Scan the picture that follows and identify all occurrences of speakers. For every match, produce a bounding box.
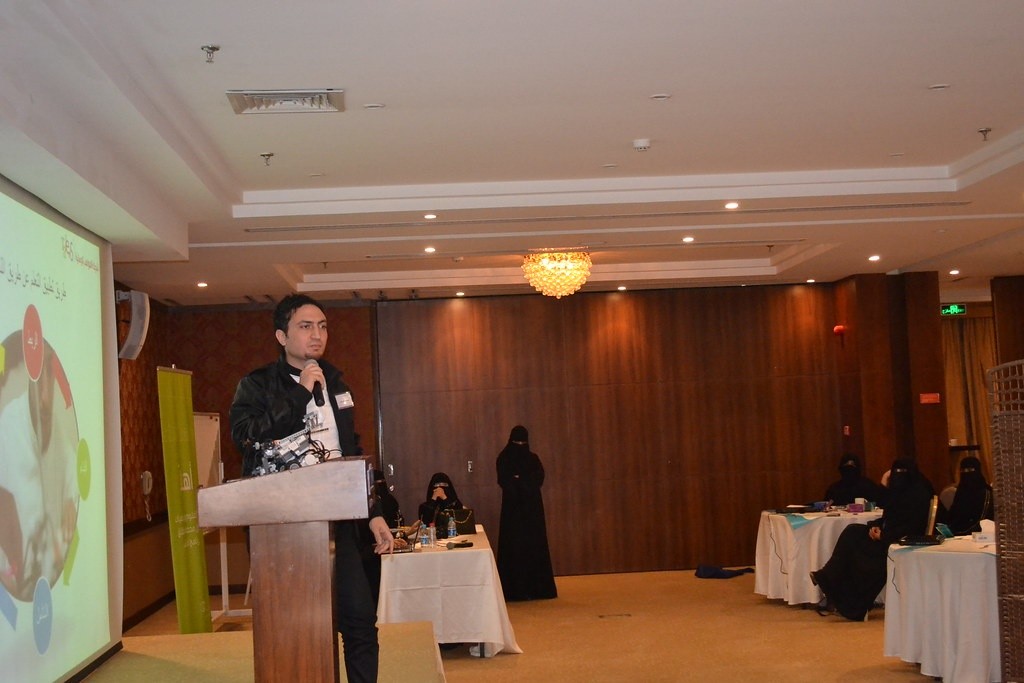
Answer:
[118,290,150,359]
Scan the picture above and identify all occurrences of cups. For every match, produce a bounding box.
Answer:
[951,439,957,446]
[854,498,864,504]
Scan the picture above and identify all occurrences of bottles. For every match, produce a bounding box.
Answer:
[428,523,436,548]
[448,517,456,538]
[420,525,429,551]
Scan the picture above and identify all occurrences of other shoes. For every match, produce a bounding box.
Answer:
[811,570,820,587]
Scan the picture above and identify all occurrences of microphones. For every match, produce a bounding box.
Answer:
[304,358,326,406]
[446,541,474,550]
[415,514,424,547]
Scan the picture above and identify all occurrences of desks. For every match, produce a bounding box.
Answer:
[375,524,523,657]
[883,532,1002,683]
[755,504,885,606]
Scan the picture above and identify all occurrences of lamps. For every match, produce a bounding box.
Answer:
[521,247,593,300]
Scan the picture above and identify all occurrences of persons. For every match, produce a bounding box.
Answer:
[496,426,558,601]
[945,456,995,537]
[828,455,886,505]
[418,473,473,651]
[229,293,396,683]
[370,469,403,529]
[810,459,937,620]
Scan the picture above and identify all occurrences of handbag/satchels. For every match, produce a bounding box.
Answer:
[443,508,477,537]
